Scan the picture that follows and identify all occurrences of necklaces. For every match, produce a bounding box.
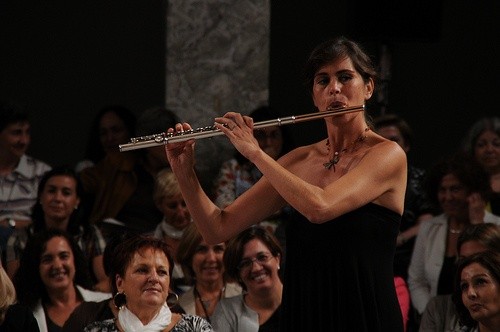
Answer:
[447,227,466,234]
[196,288,222,325]
[324,127,369,168]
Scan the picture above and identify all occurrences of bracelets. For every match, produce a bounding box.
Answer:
[398,233,406,246]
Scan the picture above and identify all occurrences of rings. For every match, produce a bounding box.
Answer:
[231,125,237,131]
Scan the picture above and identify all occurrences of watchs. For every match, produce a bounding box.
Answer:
[5,218,17,231]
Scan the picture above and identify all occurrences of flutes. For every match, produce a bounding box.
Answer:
[118,104,366,153]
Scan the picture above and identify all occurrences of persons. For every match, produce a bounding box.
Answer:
[165,38,408,332]
[0,107,500,332]
